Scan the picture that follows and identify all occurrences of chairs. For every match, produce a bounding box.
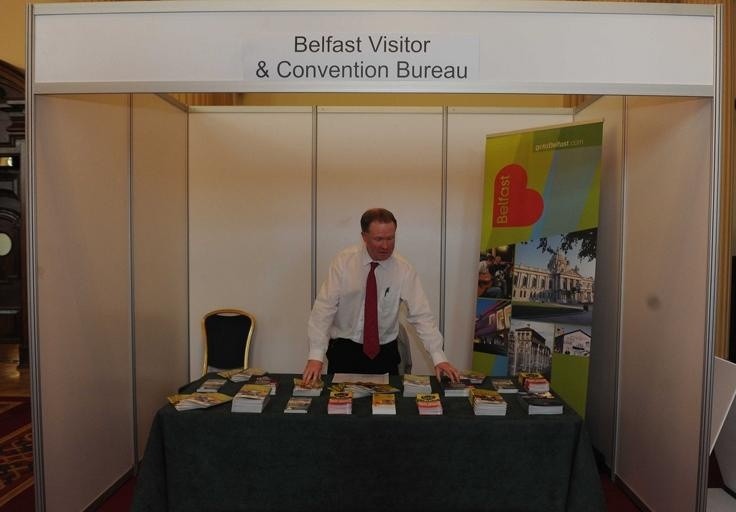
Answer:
[176,308,258,395]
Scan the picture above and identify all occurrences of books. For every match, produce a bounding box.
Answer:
[165,367,565,415]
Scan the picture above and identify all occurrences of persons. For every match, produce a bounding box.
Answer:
[301,209,461,388]
[479,254,512,299]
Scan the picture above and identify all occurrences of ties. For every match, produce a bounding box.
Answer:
[363,262,381,360]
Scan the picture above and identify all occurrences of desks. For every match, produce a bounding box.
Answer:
[155,376,583,512]
